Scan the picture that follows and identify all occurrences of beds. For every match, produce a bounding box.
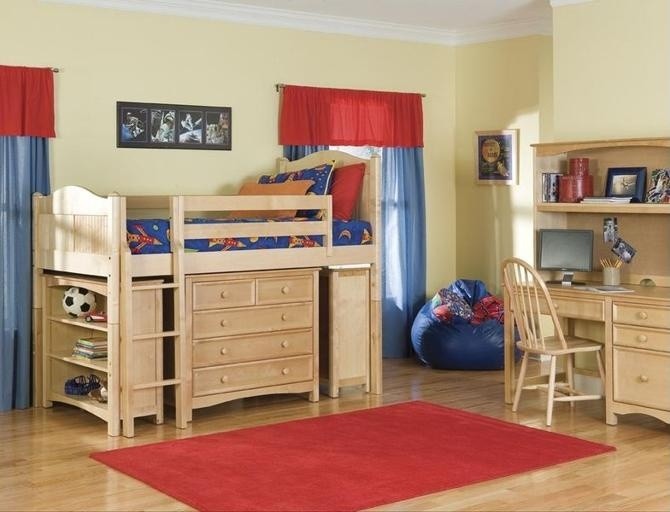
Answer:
[29,150,382,438]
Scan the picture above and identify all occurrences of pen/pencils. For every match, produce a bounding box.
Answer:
[599,256,624,269]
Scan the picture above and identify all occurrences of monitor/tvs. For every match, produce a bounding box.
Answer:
[538,228,594,287]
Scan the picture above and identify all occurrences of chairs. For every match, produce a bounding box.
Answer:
[499,256,606,425]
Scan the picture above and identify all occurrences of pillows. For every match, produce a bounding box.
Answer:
[225,159,367,222]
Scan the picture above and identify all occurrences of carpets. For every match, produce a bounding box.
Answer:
[86,400,617,512]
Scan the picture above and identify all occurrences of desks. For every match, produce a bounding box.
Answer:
[500,281,669,431]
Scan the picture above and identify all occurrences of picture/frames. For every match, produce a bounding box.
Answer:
[474,129,520,186]
[605,166,647,205]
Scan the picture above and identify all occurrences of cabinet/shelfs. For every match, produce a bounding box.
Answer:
[529,136,670,215]
[34,271,162,438]
[164,265,323,415]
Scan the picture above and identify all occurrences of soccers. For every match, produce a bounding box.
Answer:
[62,286,99,320]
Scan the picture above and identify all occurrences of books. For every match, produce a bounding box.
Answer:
[580,195,632,204]
[574,282,634,298]
[542,172,564,202]
[70,337,108,364]
